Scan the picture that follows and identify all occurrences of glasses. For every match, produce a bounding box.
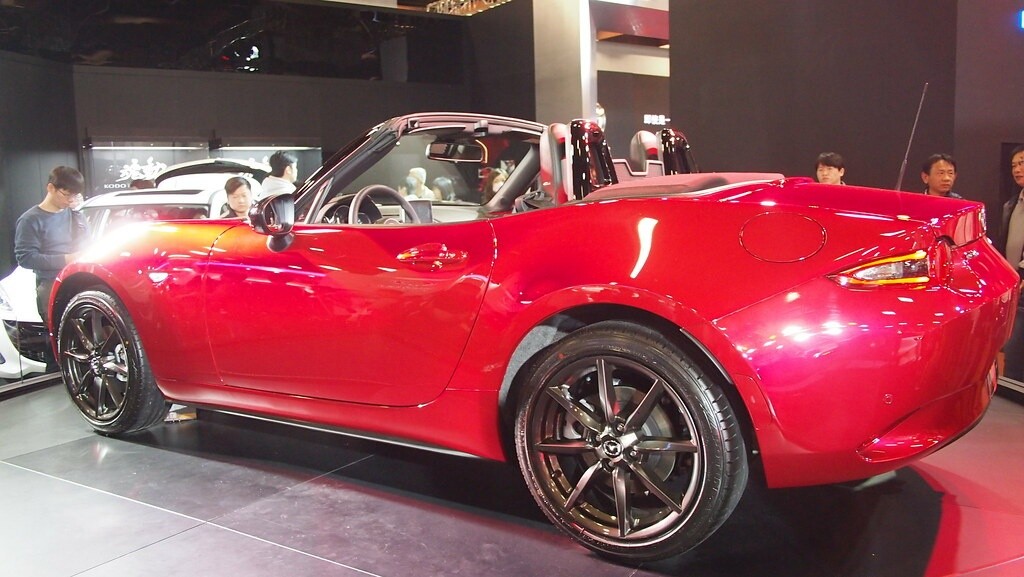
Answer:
[57,186,79,198]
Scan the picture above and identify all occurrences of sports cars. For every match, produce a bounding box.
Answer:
[44,109,1022,567]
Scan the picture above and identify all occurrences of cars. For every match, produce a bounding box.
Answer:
[0,158,272,382]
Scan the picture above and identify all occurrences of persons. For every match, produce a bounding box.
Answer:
[219,177,254,218]
[999,145,1024,298]
[257,150,298,200]
[921,153,963,199]
[129,179,153,191]
[397,167,462,201]
[815,152,846,185]
[14,165,88,372]
[485,167,510,202]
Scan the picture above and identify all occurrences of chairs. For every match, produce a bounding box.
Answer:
[514,118,619,211]
[630,128,699,176]
